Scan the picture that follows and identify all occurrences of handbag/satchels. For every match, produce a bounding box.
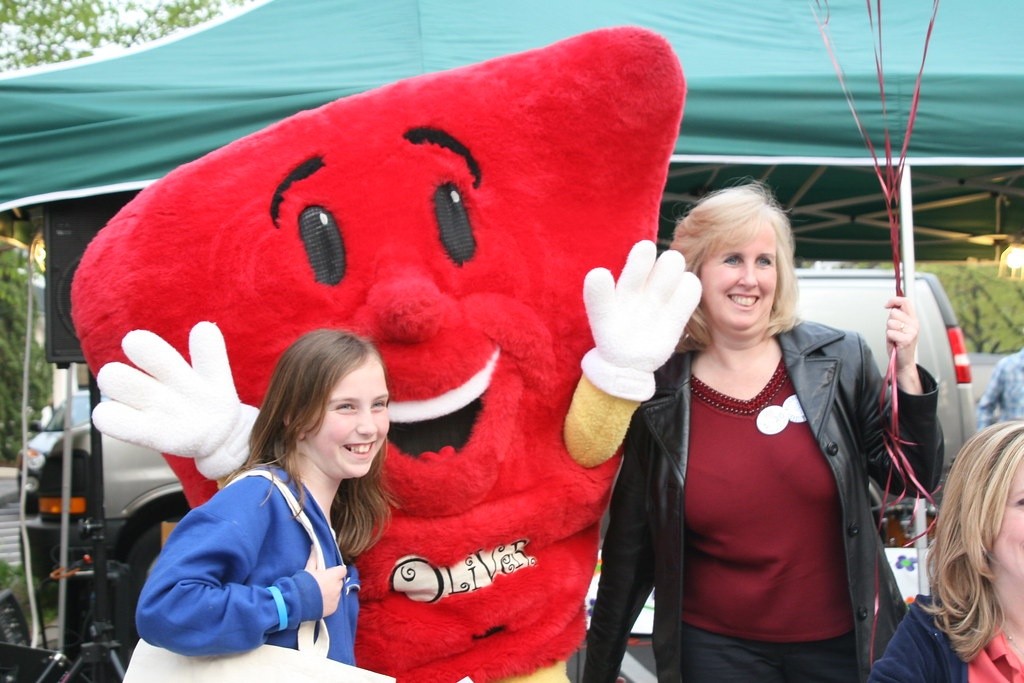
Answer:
[120,469,396,683]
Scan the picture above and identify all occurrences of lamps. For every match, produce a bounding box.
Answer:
[996,241,1024,282]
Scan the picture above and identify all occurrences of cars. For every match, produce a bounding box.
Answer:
[16,386,189,632]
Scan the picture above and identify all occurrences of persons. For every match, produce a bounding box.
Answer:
[579,183,945,683]
[976,346,1024,432]
[135,328,401,667]
[868,419,1024,683]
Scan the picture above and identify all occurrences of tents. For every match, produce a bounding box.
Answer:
[0,0,1024,652]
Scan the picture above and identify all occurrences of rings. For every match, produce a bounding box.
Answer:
[899,323,904,332]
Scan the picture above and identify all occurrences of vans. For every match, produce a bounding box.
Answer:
[789,268,982,515]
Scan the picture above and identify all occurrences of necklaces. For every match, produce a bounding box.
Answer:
[1002,628,1024,657]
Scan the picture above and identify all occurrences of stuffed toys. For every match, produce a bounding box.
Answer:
[71,25,702,683]
